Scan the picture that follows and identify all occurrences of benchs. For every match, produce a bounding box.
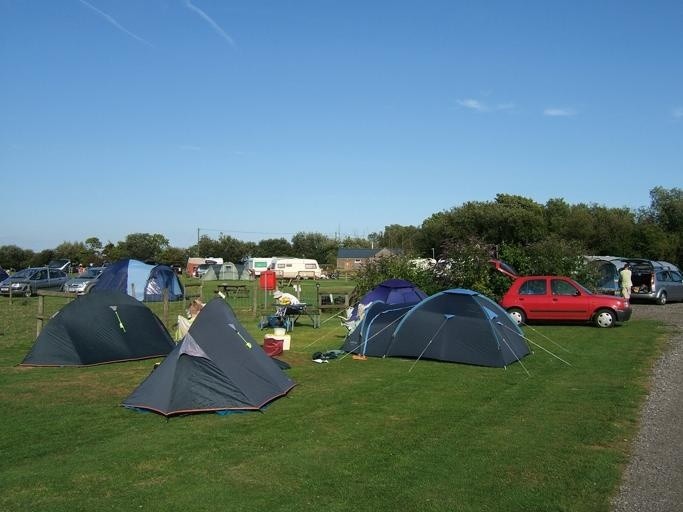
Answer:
[214,290,250,296]
[271,290,354,329]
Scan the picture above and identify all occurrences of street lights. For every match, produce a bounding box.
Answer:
[431,247,434,259]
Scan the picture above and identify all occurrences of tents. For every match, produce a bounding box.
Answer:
[120,293,298,416]
[92,257,184,303]
[340,286,531,369]
[336,275,429,334]
[12,288,177,368]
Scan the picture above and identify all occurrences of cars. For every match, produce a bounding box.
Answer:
[486,259,632,329]
[0,258,107,298]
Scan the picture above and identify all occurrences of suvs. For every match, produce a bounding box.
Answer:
[620,256,682,305]
[170,264,181,275]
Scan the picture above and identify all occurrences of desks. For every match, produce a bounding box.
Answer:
[217,284,248,298]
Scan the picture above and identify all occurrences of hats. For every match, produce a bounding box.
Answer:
[273,290,283,300]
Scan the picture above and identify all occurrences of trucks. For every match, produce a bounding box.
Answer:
[194,255,322,281]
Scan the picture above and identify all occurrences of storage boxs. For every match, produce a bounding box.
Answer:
[263,333,291,351]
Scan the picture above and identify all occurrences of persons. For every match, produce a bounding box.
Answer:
[270,288,301,313]
[619,263,634,304]
